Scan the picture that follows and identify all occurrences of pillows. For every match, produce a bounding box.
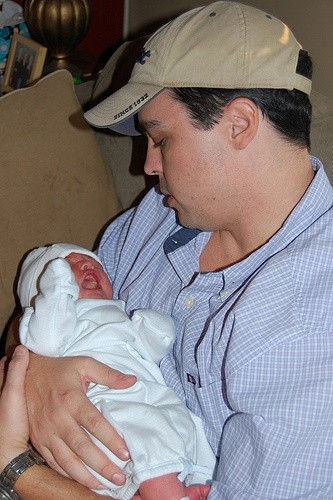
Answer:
[0,69,124,359]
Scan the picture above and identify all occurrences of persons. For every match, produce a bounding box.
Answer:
[17,242,215,500]
[0,0,333,500]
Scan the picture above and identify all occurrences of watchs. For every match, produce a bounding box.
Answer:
[0,448,46,500]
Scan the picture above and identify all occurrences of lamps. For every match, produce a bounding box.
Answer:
[24,0,99,72]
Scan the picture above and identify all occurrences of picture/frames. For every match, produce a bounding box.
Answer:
[0,33,47,94]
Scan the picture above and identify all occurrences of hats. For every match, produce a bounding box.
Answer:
[17,242,103,307]
[83,1,312,136]
